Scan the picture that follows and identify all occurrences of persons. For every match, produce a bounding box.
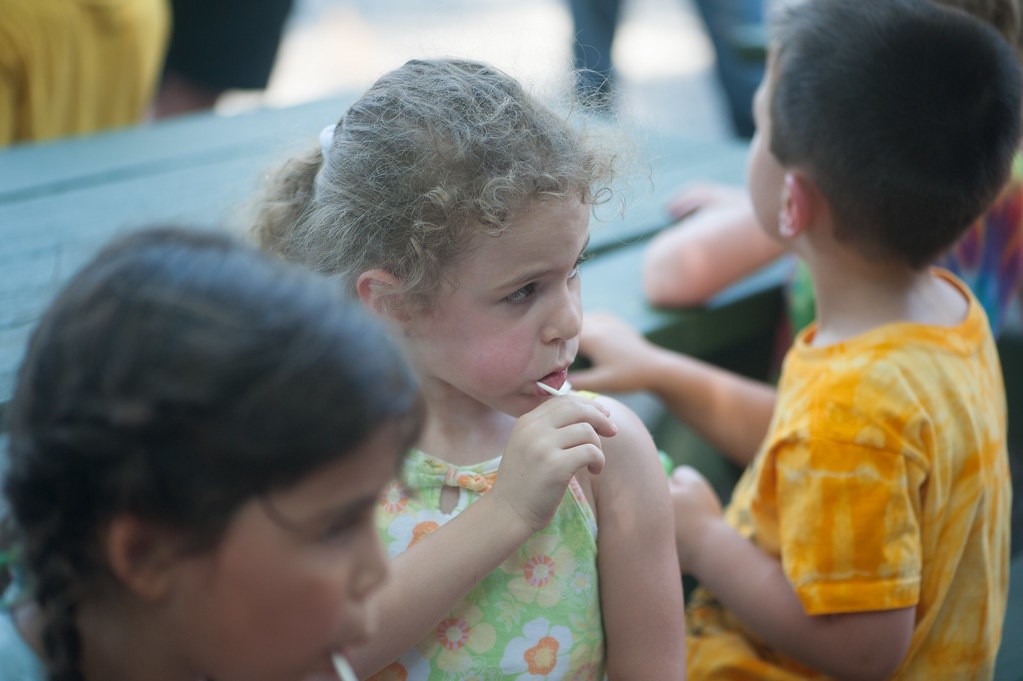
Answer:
[572,0,1023,681]
[573,0,769,139]
[1,228,431,680]
[640,0,1023,350]
[254,54,686,681]
